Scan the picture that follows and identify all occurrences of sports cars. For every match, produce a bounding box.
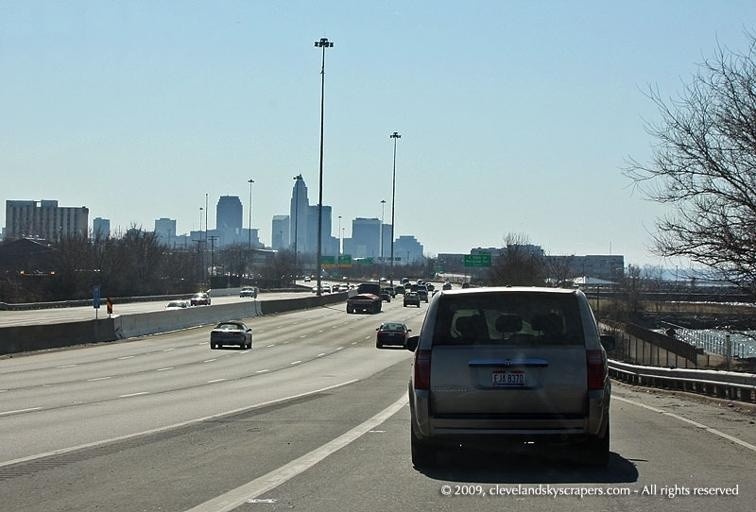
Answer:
[210,320,254,351]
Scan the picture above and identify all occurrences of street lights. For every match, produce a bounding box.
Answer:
[337,215,341,255]
[388,132,403,272]
[200,207,203,241]
[380,200,386,258]
[312,38,335,282]
[199,191,209,249]
[341,227,345,254]
[293,175,303,286]
[247,179,255,249]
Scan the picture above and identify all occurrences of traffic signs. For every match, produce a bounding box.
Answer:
[463,254,492,268]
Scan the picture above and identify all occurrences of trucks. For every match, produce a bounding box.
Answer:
[346,283,382,314]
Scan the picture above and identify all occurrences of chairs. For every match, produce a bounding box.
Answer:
[451,311,581,344]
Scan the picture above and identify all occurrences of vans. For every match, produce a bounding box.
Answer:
[400,282,611,472]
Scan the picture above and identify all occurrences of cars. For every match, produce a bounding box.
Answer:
[190,293,212,306]
[379,277,478,308]
[304,276,311,282]
[165,300,190,310]
[240,286,258,298]
[375,321,412,348]
[312,274,352,293]
